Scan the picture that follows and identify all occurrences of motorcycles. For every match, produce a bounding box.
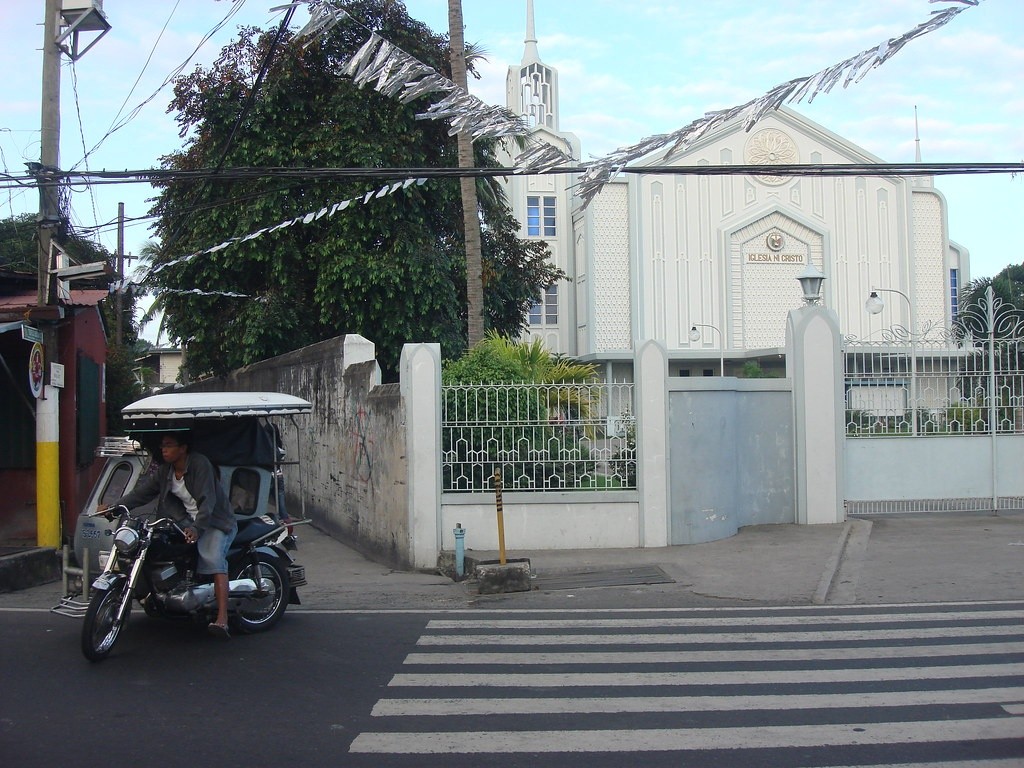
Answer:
[51,389,312,664]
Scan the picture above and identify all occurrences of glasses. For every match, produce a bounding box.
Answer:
[159,443,180,450]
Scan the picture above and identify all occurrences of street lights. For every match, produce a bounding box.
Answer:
[688,322,725,377]
[865,286,913,339]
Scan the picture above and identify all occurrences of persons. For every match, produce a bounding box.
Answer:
[265,423,289,521]
[98,429,238,639]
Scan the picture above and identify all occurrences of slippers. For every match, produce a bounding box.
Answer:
[208,623,232,639]
